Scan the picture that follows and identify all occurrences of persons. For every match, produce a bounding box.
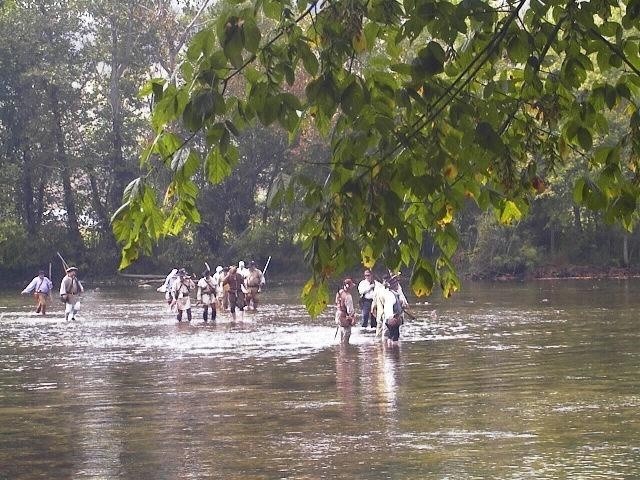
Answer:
[59,267,83,321]
[162,258,267,322]
[335,279,355,346]
[358,268,409,346]
[20,269,53,315]
